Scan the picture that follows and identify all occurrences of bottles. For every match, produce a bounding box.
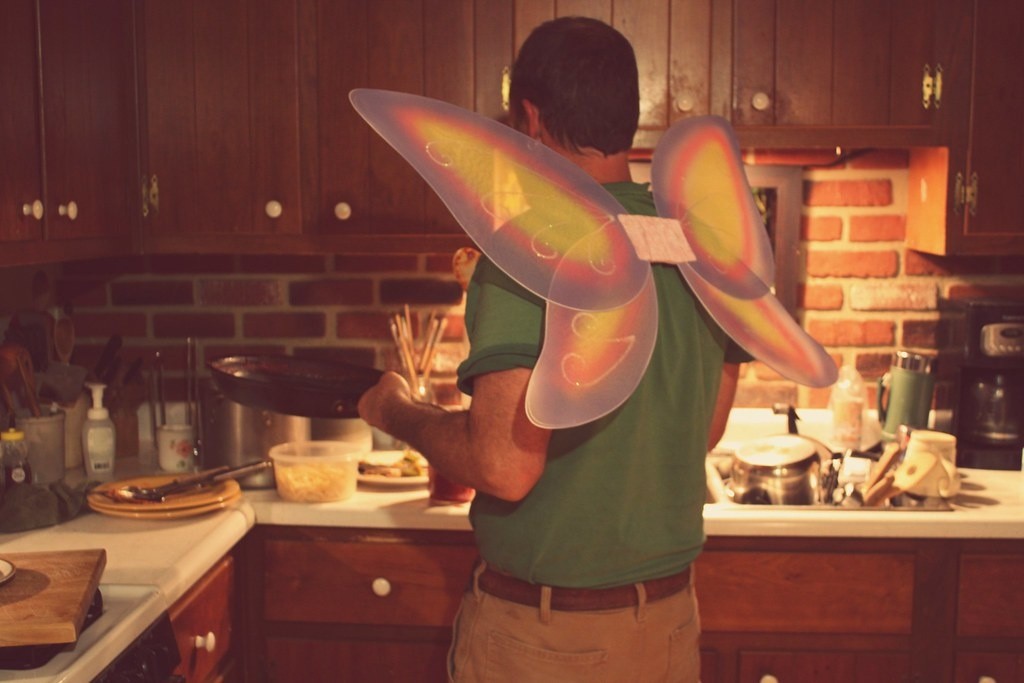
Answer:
[831,352,864,451]
[0,427,32,486]
[427,384,473,506]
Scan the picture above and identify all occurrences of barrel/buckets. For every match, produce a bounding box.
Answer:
[198,378,373,490]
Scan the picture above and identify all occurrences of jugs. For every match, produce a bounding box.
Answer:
[962,371,1024,447]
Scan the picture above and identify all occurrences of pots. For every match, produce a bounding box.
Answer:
[205,353,450,419]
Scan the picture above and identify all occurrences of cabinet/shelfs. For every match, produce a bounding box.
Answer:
[89,523,1024,683]
[0,0,1024,266]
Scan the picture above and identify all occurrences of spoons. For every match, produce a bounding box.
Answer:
[114,459,271,505]
[54,314,76,368]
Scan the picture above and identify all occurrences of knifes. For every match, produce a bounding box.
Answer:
[92,334,144,384]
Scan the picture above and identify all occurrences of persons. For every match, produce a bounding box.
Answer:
[357,16,755,683]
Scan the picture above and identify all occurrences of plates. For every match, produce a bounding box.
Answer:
[358,449,430,484]
[0,557,16,585]
[86,474,241,519]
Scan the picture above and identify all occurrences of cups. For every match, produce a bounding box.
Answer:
[892,424,961,499]
[725,435,822,505]
[15,411,66,483]
[158,423,196,472]
[49,400,87,467]
[875,350,937,436]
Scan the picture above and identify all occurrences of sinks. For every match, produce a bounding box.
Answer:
[702,459,729,503]
[704,444,956,516]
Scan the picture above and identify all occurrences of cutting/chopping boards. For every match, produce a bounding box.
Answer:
[0,548,107,647]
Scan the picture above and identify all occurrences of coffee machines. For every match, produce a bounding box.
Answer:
[929,296,1024,471]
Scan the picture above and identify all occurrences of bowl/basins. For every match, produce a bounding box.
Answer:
[267,440,360,503]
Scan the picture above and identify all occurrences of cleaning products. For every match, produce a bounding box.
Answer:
[84,382,117,480]
